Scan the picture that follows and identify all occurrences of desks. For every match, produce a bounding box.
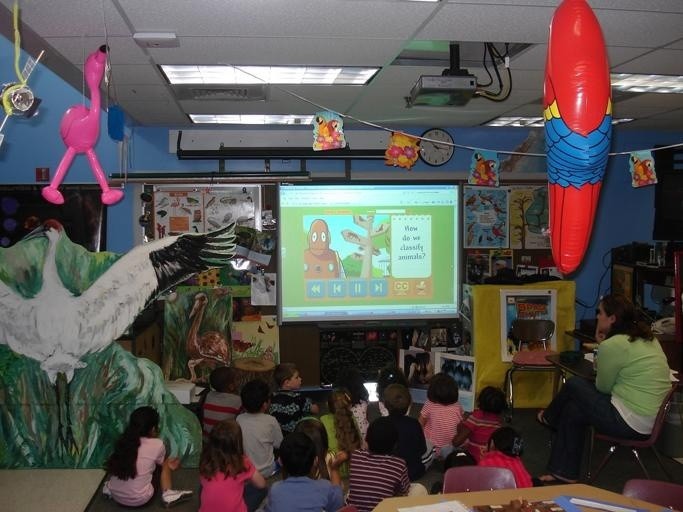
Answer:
[277,363,335,401]
[370,483,683,512]
[0,469,109,512]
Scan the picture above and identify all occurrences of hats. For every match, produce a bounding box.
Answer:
[494,427,522,456]
[442,447,474,468]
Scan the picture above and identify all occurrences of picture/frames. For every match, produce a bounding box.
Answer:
[509,186,552,250]
[462,183,510,249]
[500,288,557,364]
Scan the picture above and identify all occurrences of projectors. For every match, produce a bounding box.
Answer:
[409,68,478,108]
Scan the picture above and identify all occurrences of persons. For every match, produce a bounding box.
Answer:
[362,384,426,484]
[201,367,246,441]
[102,406,193,509]
[319,386,366,484]
[343,416,411,512]
[266,432,348,512]
[478,427,534,489]
[265,362,319,438]
[198,419,266,512]
[416,373,464,458]
[452,386,509,465]
[294,419,340,485]
[338,373,371,451]
[235,378,287,481]
[366,365,420,421]
[532,293,669,484]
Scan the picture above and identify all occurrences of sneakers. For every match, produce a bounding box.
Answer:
[101,482,111,498]
[163,490,194,506]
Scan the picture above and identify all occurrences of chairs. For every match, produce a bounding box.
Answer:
[504,319,566,424]
[587,383,678,482]
[622,478,683,512]
[443,466,517,493]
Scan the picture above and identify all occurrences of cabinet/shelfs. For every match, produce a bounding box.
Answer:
[318,319,463,388]
[634,260,675,319]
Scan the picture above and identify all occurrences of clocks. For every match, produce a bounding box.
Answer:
[417,127,455,167]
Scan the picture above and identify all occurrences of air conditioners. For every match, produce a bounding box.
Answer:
[170,83,270,102]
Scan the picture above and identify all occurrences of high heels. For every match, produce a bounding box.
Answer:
[536,408,557,433]
